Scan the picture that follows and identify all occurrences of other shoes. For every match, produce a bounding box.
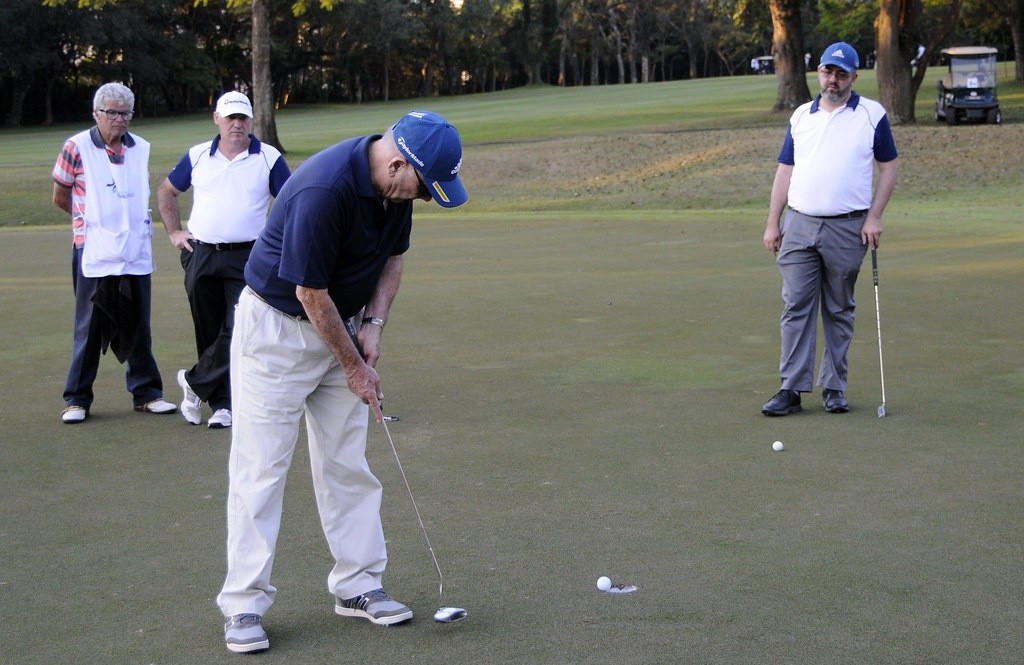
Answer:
[177,369,204,425]
[207,408,232,428]
[134,398,177,414]
[60,405,90,424]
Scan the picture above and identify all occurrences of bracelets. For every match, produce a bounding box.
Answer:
[360,316,385,329]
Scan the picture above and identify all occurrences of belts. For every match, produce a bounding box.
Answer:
[817,209,870,218]
[192,240,256,251]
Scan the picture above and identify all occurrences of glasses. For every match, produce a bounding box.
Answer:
[99,109,134,121]
[412,168,432,202]
[821,68,850,80]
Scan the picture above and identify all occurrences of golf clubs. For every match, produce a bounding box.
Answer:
[871,242,886,419]
[343,312,470,624]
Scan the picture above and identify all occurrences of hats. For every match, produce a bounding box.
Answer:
[392,109,470,208]
[216,91,254,119]
[818,42,860,73]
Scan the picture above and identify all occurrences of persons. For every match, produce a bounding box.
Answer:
[761,42,899,415]
[217,109,468,652]
[50,83,176,423]
[158,91,290,427]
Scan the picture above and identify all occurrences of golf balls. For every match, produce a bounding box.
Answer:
[596,575,611,592]
[773,440,784,451]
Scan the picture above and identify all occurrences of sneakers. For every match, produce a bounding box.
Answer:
[822,388,850,413]
[335,588,414,625]
[224,613,269,652]
[761,389,802,415]
